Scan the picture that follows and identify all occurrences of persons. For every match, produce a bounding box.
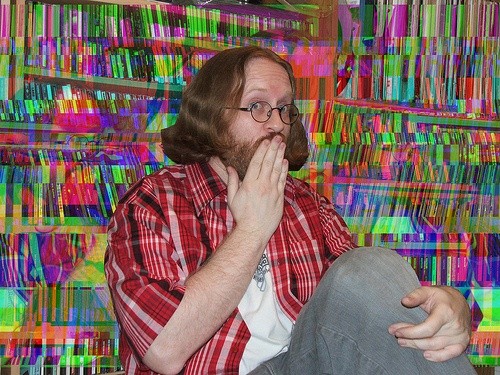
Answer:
[104,46,483,375]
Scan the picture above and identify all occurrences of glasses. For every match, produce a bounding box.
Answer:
[224,101,299,125]
